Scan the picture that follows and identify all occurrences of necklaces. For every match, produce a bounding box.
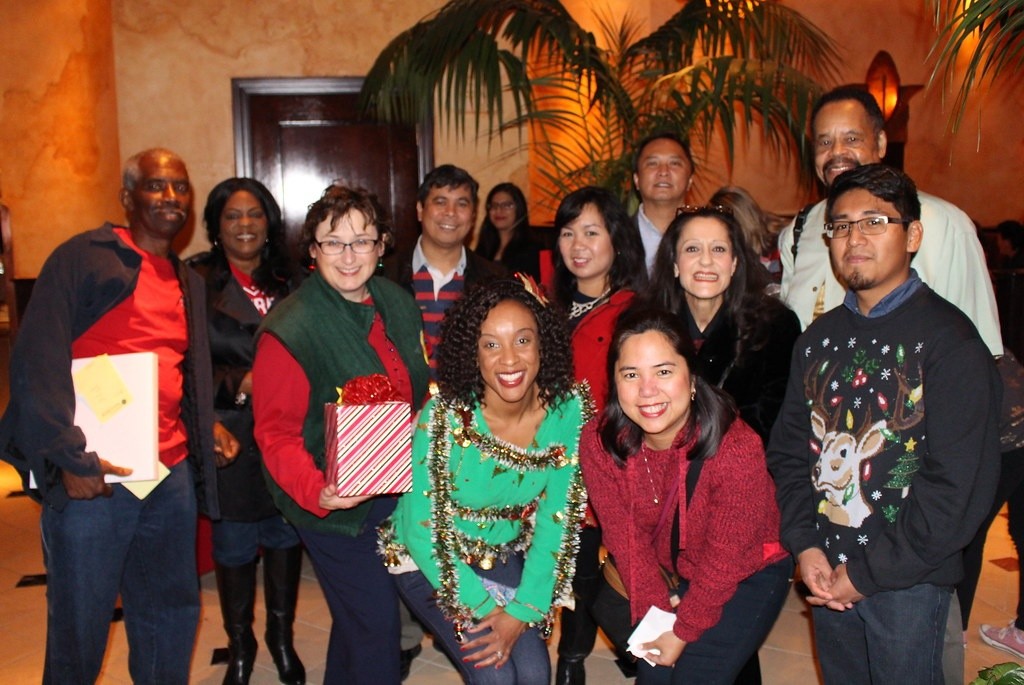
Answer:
[641,436,659,504]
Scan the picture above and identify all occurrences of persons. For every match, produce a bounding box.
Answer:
[0,83,1024,685]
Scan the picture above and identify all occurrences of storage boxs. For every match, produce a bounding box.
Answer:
[26,351,161,490]
[324,401,414,497]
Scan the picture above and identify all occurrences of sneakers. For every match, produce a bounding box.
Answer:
[978,617,1024,660]
[963,631,967,648]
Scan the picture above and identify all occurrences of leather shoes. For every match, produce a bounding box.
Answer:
[556,655,585,685]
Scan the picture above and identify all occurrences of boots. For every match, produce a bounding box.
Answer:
[213,555,260,685]
[261,541,306,685]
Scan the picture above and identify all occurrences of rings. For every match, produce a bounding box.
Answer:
[496,650,503,659]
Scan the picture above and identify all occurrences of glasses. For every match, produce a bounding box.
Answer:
[312,234,380,255]
[487,201,515,210]
[673,205,734,228]
[823,216,910,239]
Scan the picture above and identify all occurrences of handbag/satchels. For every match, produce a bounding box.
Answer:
[993,347,1024,453]
[591,550,681,663]
